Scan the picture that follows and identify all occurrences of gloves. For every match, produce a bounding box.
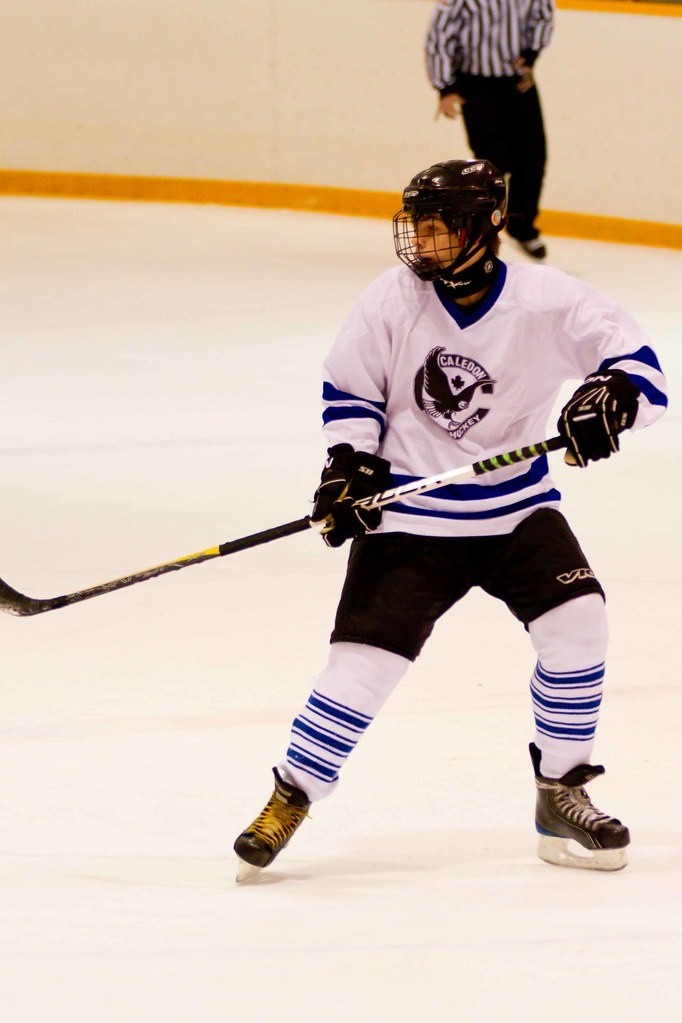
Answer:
[311,443,391,548]
[557,369,640,469]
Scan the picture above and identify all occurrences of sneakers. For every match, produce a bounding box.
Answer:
[529,742,630,870]
[234,766,312,883]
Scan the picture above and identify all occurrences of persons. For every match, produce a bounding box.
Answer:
[423,0,556,266]
[233,160,667,885]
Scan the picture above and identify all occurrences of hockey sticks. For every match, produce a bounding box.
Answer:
[0,435,566,617]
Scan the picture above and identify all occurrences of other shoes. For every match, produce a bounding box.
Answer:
[520,235,546,258]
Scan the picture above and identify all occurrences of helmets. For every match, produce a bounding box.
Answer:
[392,159,507,282]
